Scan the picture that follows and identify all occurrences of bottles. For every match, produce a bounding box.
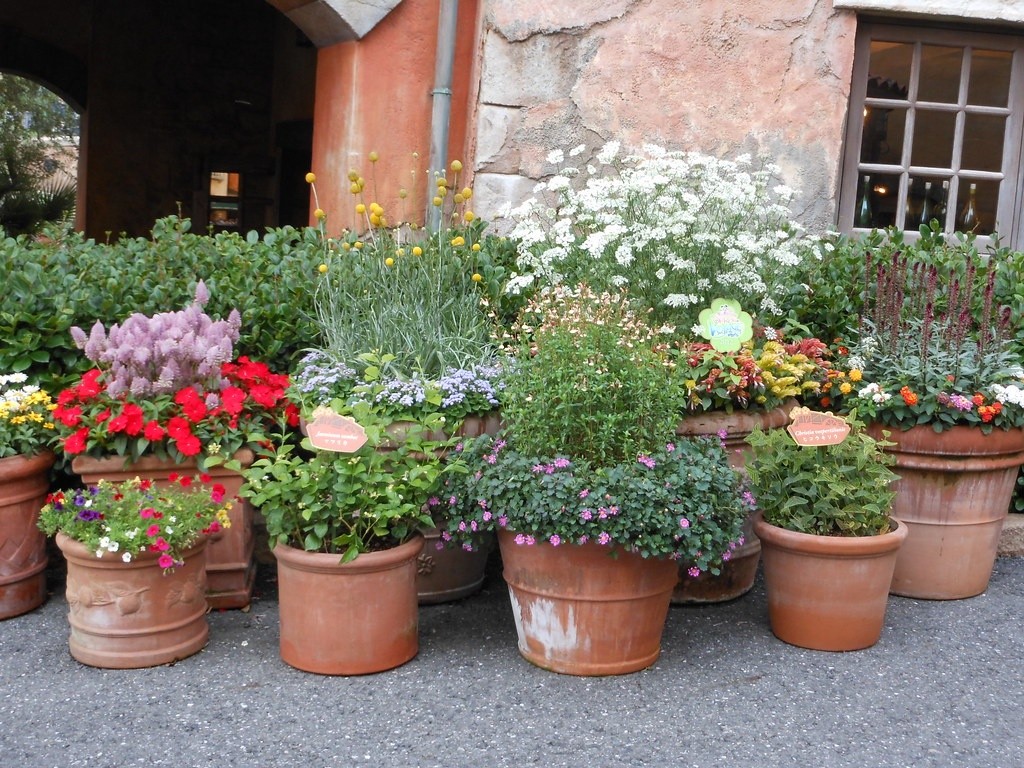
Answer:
[957,183,981,236]
[903,177,916,232]
[917,182,936,231]
[854,174,873,229]
[935,181,950,232]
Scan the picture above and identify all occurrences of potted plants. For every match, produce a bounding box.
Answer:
[744,407,908,654]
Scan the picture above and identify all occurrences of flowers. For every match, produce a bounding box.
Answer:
[0,372,58,460]
[47,278,299,472]
[653,322,859,409]
[36,468,243,576]
[826,249,1024,433]
[284,154,515,430]
[228,355,468,563]
[438,284,754,583]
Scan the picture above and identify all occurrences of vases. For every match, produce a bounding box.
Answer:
[859,422,1024,601]
[54,532,209,670]
[295,413,504,605]
[0,452,60,621]
[71,455,258,608]
[493,524,681,676]
[639,401,801,604]
[269,538,423,676]
